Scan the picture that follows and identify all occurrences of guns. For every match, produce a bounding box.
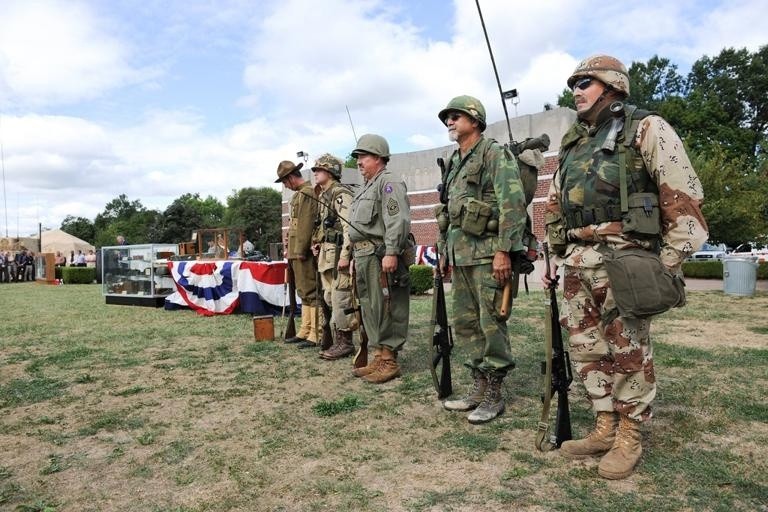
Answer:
[285,259,302,339]
[543,242,573,448]
[344,260,368,368]
[313,246,334,350]
[434,242,453,400]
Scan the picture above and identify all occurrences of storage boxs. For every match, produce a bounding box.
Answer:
[253,315,275,342]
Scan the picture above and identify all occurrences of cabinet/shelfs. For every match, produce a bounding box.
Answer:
[102,243,180,308]
[34,252,55,285]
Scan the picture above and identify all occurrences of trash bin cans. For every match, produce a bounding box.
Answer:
[720,255,760,296]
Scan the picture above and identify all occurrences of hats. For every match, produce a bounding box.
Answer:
[274,160,303,183]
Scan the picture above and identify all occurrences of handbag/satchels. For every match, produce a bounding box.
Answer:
[602,247,679,319]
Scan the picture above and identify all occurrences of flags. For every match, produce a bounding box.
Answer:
[412,245,453,273]
[537,240,545,257]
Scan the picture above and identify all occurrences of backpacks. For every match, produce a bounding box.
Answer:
[442,138,538,299]
[315,183,363,234]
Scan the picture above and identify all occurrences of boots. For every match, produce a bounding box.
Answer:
[322,327,355,360]
[350,348,381,377]
[361,348,401,383]
[444,378,488,411]
[598,414,642,479]
[318,328,342,358]
[468,380,504,423]
[559,411,619,459]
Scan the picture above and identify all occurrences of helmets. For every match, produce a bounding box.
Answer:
[311,155,342,183]
[351,134,391,161]
[438,96,487,132]
[567,55,630,97]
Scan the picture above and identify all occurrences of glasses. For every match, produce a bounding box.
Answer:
[444,114,460,124]
[573,76,591,90]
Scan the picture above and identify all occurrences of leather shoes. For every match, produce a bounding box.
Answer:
[296,339,316,348]
[284,337,302,343]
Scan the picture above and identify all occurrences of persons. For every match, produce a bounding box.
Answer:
[228,239,264,260]
[432,93,529,424]
[205,239,219,253]
[348,133,410,385]
[539,54,712,480]
[272,159,326,350]
[310,153,360,361]
[117,235,129,277]
[0,248,96,284]
